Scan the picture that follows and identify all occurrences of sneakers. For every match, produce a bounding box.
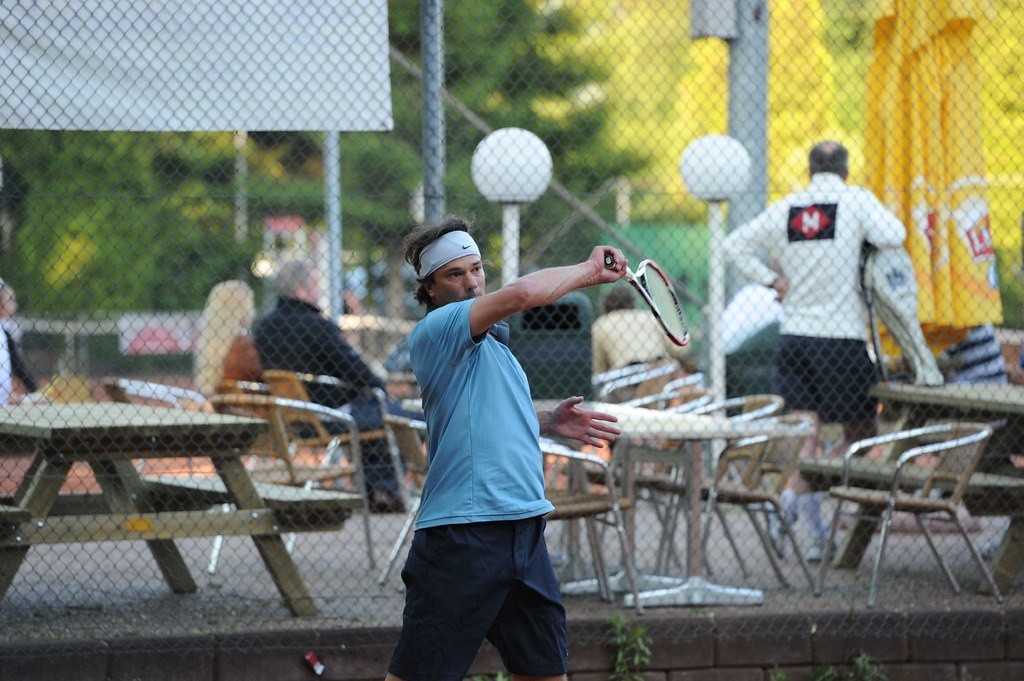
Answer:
[761,496,835,564]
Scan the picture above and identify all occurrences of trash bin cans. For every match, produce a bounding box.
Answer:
[502,290,593,401]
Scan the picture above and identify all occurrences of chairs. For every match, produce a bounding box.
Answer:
[0,356,1023,622]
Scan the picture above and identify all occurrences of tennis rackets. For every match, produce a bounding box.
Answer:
[604,254,691,345]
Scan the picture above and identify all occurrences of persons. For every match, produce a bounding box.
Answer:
[373,215,631,680]
[247,255,414,515]
[588,285,674,398]
[713,137,911,560]
[0,278,34,403]
